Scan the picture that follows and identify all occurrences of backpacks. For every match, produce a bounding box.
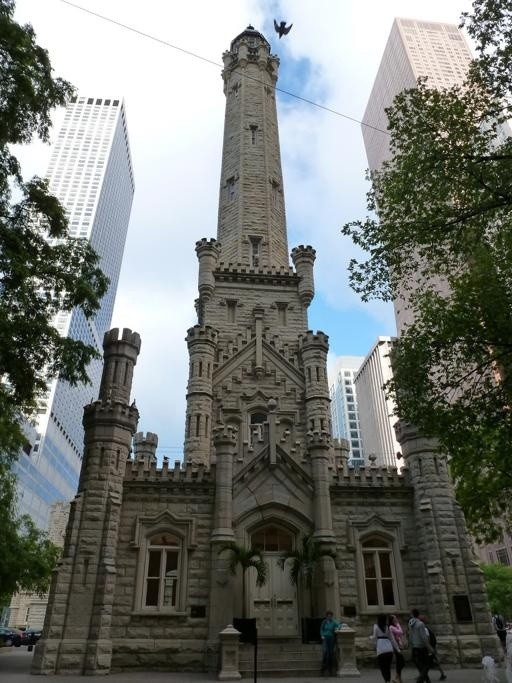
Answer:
[494,614,503,629]
[424,624,437,648]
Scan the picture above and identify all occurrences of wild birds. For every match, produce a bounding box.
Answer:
[274,19,293,39]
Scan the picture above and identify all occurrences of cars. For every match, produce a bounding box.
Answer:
[0,626,43,647]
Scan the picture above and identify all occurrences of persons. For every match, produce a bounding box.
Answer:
[407,607,436,682]
[368,612,401,683]
[416,614,447,680]
[388,614,406,682]
[319,609,342,676]
[492,609,507,655]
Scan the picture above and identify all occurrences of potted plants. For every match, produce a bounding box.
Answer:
[277,528,342,644]
[217,541,268,643]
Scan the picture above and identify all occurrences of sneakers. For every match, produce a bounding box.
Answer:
[383,675,447,683]
